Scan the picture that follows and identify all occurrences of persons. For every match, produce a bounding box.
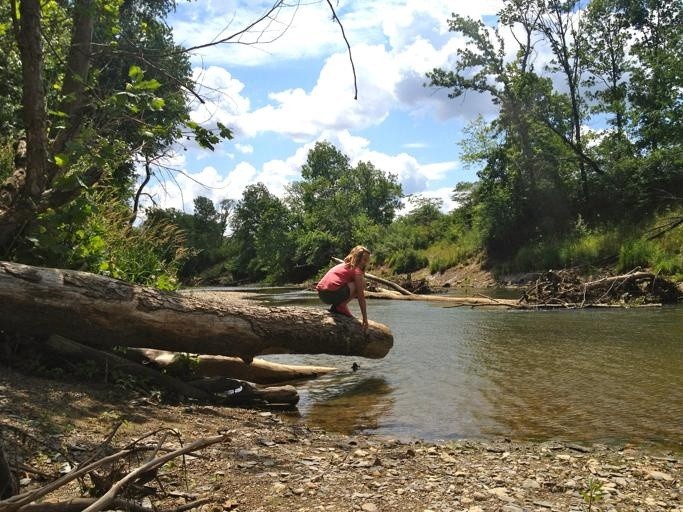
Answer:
[316,245,369,328]
